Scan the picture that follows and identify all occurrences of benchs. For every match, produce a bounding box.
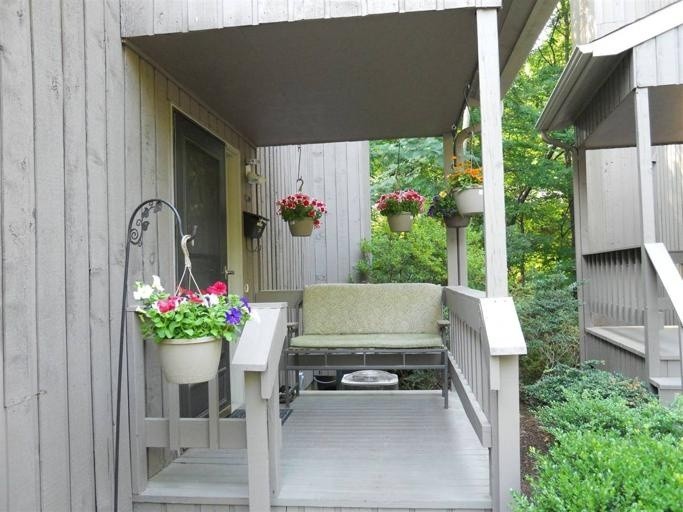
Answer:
[284,282,447,413]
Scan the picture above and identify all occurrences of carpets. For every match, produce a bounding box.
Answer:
[227,407,293,426]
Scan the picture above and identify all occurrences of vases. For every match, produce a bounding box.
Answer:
[450,183,485,215]
[385,210,414,233]
[444,211,471,228]
[287,216,315,238]
[152,333,222,386]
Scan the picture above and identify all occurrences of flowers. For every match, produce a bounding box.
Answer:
[425,185,473,220]
[442,155,484,196]
[374,189,429,216]
[130,273,251,342]
[276,193,330,227]
